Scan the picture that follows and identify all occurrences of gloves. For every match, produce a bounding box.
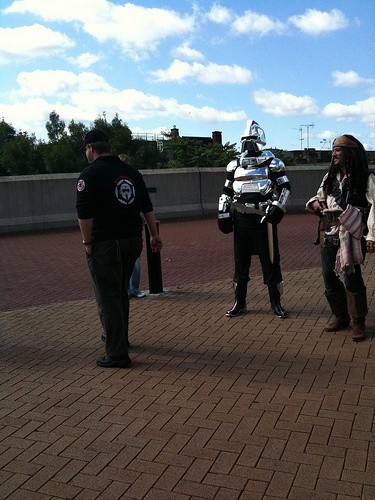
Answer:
[217,212,234,234]
[266,205,285,224]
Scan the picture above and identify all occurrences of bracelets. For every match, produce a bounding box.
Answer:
[82,238,93,246]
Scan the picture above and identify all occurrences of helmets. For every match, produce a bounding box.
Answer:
[239,120,266,153]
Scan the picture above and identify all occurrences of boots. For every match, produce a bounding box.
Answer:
[346,286,367,342]
[266,281,288,318]
[225,280,247,316]
[323,281,350,332]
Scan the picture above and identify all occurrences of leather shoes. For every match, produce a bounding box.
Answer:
[102,331,131,348]
[97,355,132,368]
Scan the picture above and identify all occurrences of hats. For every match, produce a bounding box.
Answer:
[79,130,108,152]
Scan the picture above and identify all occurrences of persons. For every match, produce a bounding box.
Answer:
[75,130,163,368]
[217,121,292,318]
[305,135,375,342]
[117,154,146,299]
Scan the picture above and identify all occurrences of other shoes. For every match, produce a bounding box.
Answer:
[130,292,146,298]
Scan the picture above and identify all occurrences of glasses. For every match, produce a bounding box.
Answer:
[83,145,89,153]
